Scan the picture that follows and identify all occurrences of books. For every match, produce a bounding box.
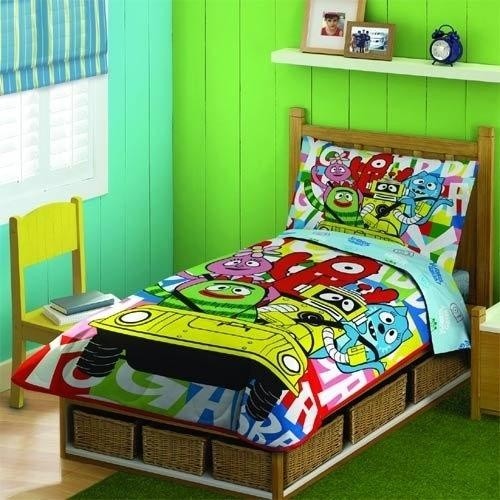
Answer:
[38,293,122,326]
[49,288,115,315]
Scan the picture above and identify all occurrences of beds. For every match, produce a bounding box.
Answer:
[60,105,495,500]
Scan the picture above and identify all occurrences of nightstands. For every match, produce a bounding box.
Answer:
[471,301,500,421]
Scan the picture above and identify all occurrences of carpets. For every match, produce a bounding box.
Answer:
[63,379,500,500]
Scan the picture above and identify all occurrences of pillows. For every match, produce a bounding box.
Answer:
[284,135,480,275]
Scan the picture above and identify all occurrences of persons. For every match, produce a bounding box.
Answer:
[349,29,370,53]
[321,12,344,37]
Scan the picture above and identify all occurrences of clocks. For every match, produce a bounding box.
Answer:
[430,25,463,67]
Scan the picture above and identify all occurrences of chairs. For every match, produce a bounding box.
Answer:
[9,196,86,408]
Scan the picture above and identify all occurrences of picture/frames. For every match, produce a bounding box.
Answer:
[301,0,366,56]
[344,21,396,62]
